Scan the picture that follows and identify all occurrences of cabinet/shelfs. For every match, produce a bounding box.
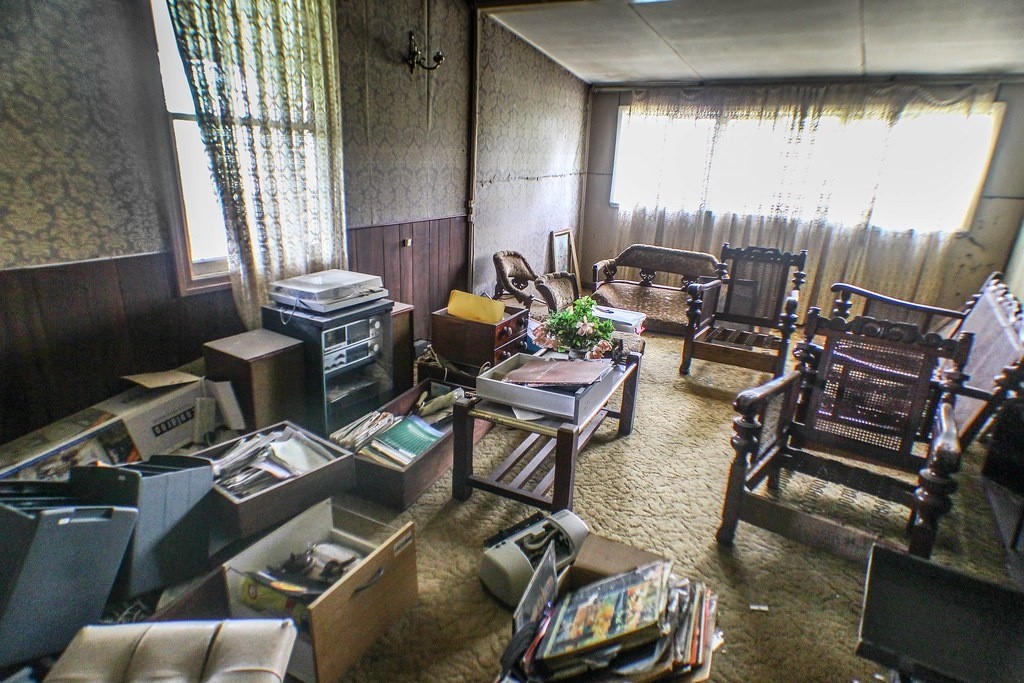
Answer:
[392,301,415,393]
[203,329,310,435]
[261,297,394,437]
[431,305,529,368]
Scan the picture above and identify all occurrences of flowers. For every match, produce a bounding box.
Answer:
[535,296,614,360]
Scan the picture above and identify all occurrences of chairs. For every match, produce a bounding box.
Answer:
[494,249,580,322]
[715,284,975,564]
[679,241,808,381]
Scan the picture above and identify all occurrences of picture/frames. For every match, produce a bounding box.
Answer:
[551,230,582,295]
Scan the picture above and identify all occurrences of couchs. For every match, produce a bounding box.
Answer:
[591,243,728,335]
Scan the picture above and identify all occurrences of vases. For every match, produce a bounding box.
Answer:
[568,348,588,360]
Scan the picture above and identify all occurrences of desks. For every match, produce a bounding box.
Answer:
[855,544,1024,683]
[451,347,642,515]
[417,358,480,389]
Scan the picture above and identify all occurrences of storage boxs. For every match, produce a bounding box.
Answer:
[476,352,610,426]
[0,356,246,482]
[0,377,495,683]
[497,532,719,683]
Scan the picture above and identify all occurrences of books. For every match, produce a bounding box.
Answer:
[500,357,615,388]
[329,408,446,469]
[212,422,338,500]
[511,539,722,683]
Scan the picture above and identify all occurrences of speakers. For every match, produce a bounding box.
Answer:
[203,328,313,433]
[391,302,416,395]
